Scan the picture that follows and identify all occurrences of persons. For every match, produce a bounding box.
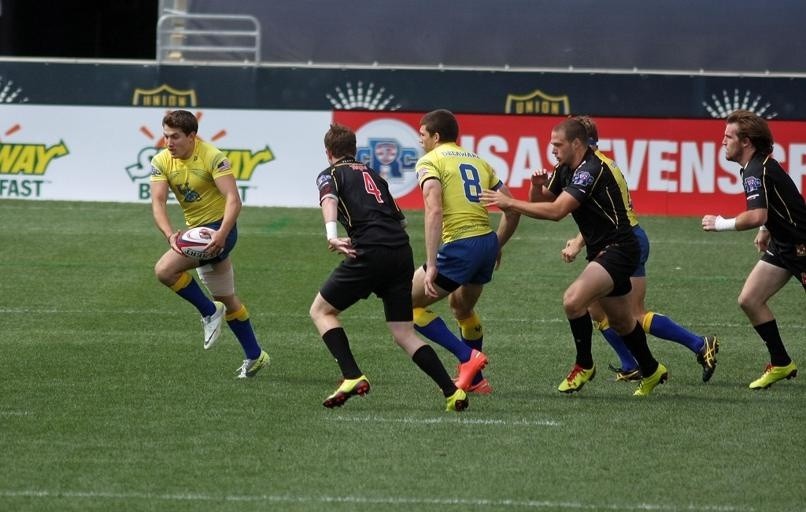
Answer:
[559,114,721,385]
[700,112,806,392]
[308,124,466,414]
[474,119,671,400]
[152,111,272,379]
[402,109,520,397]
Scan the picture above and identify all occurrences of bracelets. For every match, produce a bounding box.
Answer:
[326,221,338,242]
[714,215,739,233]
[166,232,175,245]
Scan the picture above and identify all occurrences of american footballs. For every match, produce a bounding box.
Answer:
[176,226,225,260]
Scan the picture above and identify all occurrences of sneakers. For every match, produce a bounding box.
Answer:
[632,362,669,397]
[605,366,643,384]
[321,374,371,409]
[696,333,722,384]
[237,349,272,380]
[201,300,228,350]
[556,358,598,393]
[465,377,495,397]
[451,348,490,392]
[748,359,798,391]
[444,387,469,413]
